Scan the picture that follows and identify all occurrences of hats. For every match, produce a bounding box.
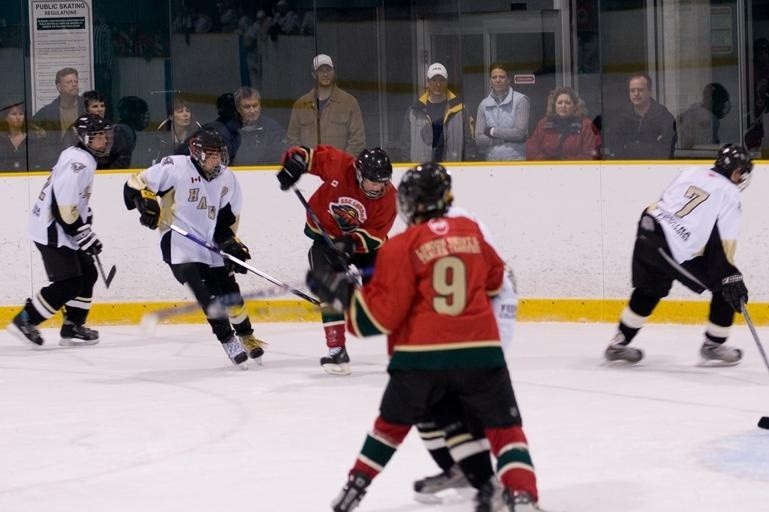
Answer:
[427,62,448,80]
[313,54,334,71]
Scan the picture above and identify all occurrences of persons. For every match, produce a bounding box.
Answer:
[406,162,520,512]
[13,115,114,347]
[148,100,201,164]
[122,126,265,366]
[0,67,121,171]
[399,63,531,160]
[675,81,731,151]
[744,77,769,160]
[601,141,757,364]
[330,159,543,512]
[220,86,284,165]
[289,55,365,158]
[275,142,397,367]
[527,73,678,161]
[166,0,316,49]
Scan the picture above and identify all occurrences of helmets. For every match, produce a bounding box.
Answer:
[714,143,753,191]
[186,128,230,182]
[397,162,453,225]
[702,82,731,119]
[118,93,150,132]
[71,114,116,160]
[216,92,248,129]
[353,146,392,200]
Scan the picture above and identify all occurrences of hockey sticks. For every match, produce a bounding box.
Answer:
[95,253,117,289]
[738,293,769,429]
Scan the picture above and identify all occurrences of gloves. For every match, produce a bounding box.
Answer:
[321,237,355,272]
[134,188,160,229]
[86,207,92,224]
[220,236,251,274]
[277,152,307,190]
[72,223,102,256]
[720,264,748,313]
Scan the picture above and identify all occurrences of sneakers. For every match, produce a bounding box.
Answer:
[605,344,642,362]
[701,339,740,362]
[414,463,469,493]
[759,414,768,430]
[235,329,264,358]
[474,474,508,512]
[501,486,544,512]
[217,330,248,364]
[321,346,349,364]
[13,309,43,345]
[331,470,372,512]
[60,324,98,340]
